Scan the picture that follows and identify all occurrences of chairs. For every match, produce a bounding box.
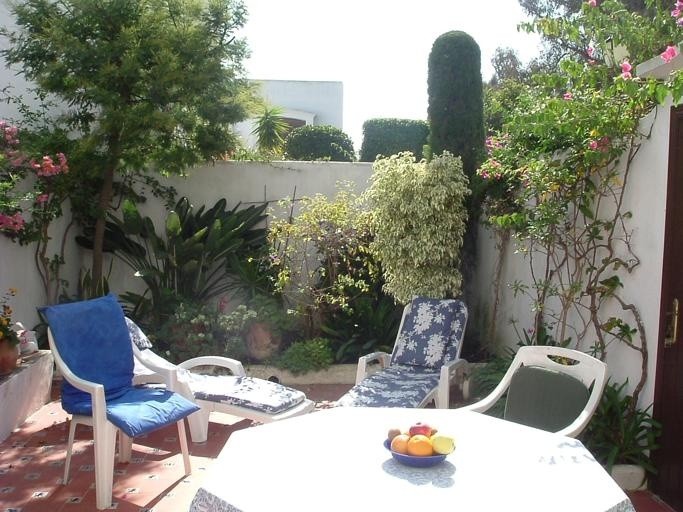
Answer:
[125,317,315,443]
[456,346,608,438]
[36,292,201,511]
[333,294,470,409]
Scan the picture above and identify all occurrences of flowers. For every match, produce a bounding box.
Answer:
[0,288,20,348]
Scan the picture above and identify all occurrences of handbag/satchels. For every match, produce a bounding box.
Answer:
[10,322,39,355]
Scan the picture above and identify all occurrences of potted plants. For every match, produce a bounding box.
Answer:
[205,248,283,361]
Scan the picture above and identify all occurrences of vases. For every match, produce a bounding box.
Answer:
[0,338,18,376]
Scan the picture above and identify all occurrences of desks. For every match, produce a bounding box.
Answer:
[190,406,634,512]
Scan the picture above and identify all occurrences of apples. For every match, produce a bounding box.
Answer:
[408,421,433,437]
[409,434,432,456]
[432,433,456,454]
[389,435,408,456]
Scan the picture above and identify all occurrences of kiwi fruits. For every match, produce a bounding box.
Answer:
[387,427,401,445]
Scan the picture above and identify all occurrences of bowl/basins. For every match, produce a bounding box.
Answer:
[384,440,445,466]
